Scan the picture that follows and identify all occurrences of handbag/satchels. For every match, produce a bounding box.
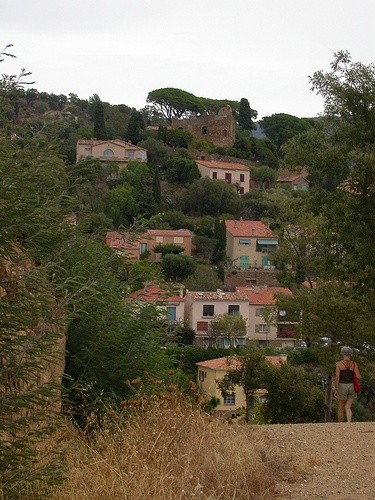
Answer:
[351,360,361,394]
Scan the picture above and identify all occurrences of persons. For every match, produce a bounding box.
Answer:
[333,347,362,423]
